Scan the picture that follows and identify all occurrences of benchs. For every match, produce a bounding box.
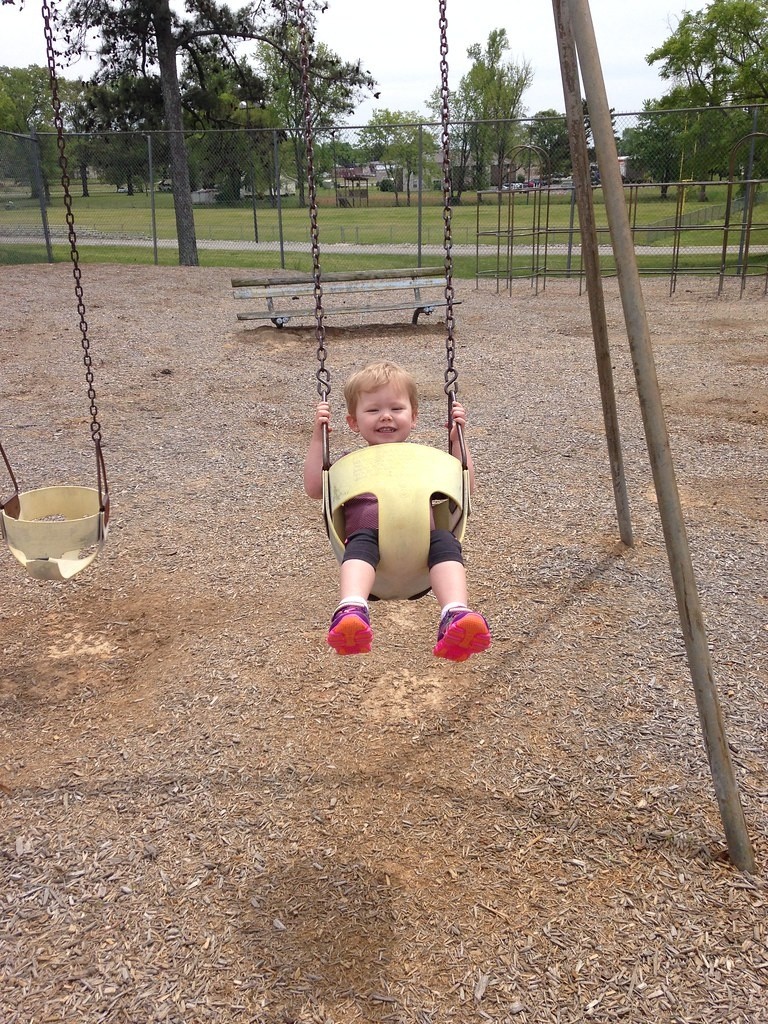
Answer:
[229,264,463,329]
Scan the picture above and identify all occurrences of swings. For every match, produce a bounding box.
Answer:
[0,0,111,583]
[296,0,470,600]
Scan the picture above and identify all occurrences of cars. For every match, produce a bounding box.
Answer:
[502,176,574,190]
[347,174,369,181]
[116,187,129,193]
[156,181,172,191]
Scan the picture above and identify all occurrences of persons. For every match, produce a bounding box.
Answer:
[305,360,489,661]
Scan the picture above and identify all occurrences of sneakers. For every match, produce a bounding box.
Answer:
[327,601,374,655]
[432,606,492,663]
